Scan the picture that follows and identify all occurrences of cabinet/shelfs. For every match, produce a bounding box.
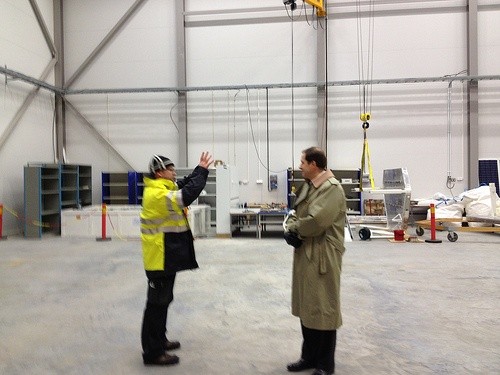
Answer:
[102,168,216,236]
[287,169,362,215]
[24,164,92,240]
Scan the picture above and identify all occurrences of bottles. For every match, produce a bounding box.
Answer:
[291,183,296,193]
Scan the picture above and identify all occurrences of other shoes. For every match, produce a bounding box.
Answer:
[165,340,181,349]
[150,352,178,364]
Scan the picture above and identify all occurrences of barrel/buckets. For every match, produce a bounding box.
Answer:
[394,230,406,241]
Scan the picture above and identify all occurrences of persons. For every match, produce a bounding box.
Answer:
[284,147,346,375]
[139,151,214,365]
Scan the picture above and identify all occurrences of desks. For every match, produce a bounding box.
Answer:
[258,210,289,240]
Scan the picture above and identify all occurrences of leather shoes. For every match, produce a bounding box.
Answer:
[313,369,327,375]
[287,361,310,371]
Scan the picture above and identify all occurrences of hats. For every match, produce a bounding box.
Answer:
[150,155,175,176]
